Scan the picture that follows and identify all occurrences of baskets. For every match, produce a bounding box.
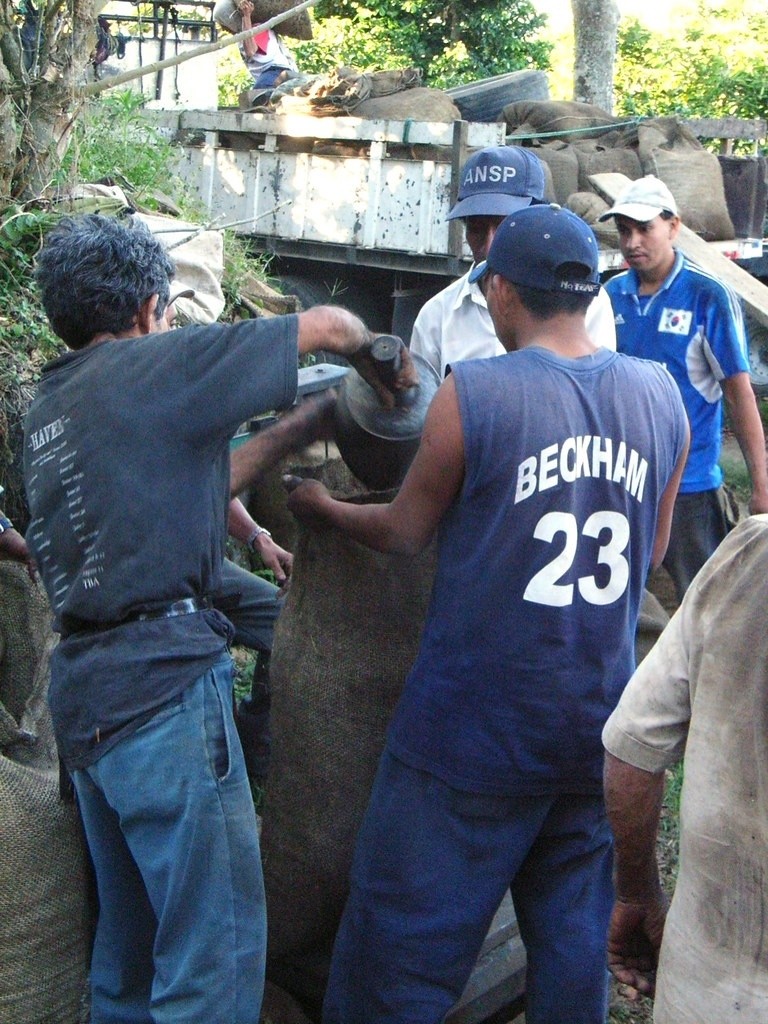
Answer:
[60,594,214,642]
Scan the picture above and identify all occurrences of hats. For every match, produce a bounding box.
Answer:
[469,202,602,297]
[444,144,545,221]
[166,279,193,309]
[597,173,678,222]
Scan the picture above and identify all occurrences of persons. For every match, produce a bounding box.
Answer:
[0,486,41,589]
[409,145,670,672]
[20,212,420,1023]
[597,169,767,611]
[316,201,692,1024]
[598,510,768,1024]
[162,284,297,783]
[217,0,300,107]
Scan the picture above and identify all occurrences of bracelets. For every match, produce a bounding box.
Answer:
[246,526,272,554]
